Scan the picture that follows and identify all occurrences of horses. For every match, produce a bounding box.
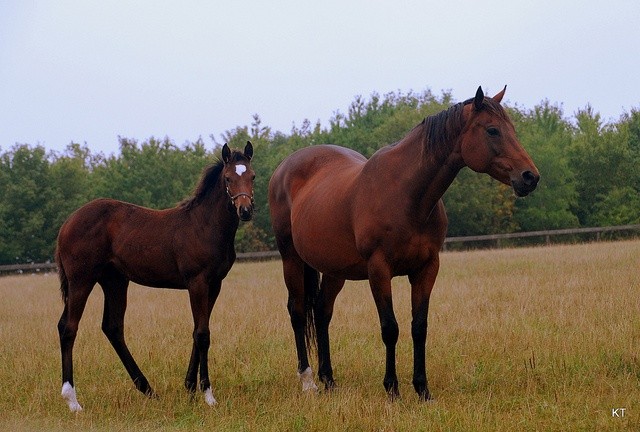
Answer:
[269,84,541,404]
[54,139,257,412]
